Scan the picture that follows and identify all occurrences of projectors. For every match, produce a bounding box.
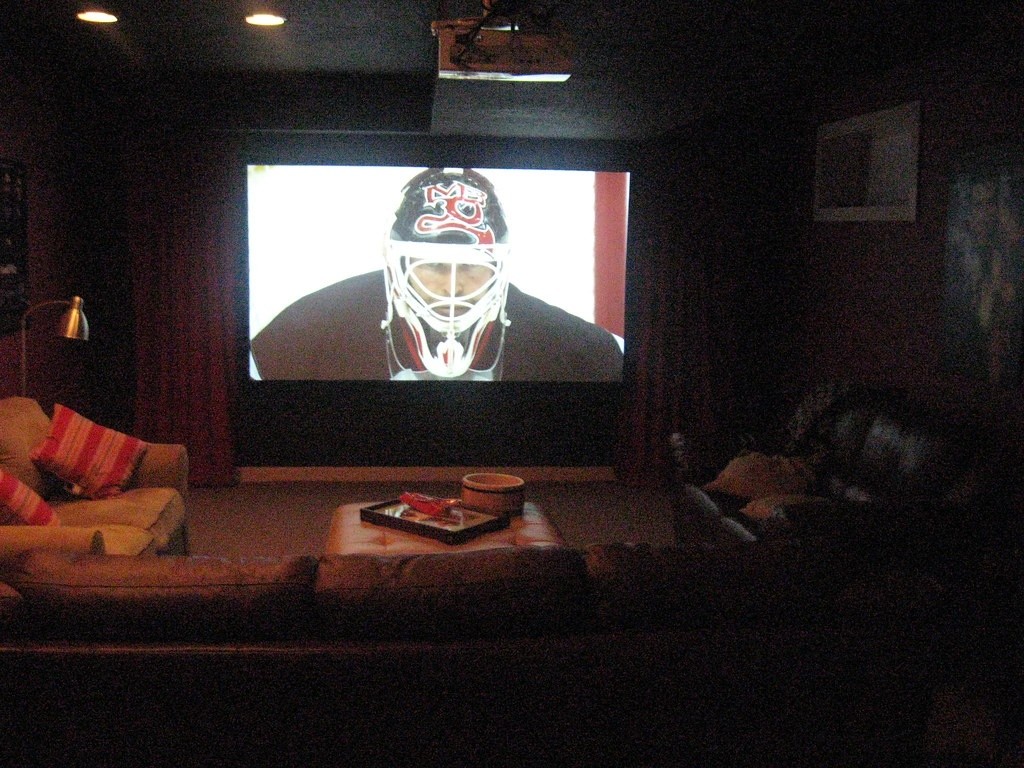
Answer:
[435,26,573,83]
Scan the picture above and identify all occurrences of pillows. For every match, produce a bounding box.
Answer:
[0,471,58,528]
[740,497,855,533]
[29,403,148,502]
[702,447,824,498]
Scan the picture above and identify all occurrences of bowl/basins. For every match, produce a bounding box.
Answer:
[461,473,525,517]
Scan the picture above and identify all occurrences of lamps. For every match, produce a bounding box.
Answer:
[21,296,89,396]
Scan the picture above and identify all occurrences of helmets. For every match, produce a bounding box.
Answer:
[384,168,512,378]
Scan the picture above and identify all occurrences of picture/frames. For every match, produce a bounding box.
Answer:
[0,157,29,335]
[812,99,923,222]
[360,500,510,545]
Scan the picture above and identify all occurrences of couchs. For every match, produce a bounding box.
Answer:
[0,383,1024,768]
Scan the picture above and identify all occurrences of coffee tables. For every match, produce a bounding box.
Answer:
[328,498,568,555]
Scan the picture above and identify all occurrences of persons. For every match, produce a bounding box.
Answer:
[248,166,624,381]
